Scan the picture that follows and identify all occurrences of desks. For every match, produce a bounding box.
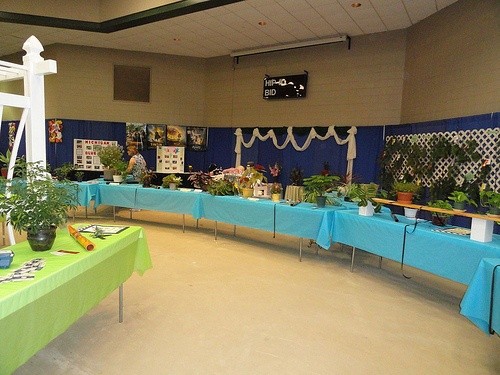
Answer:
[459,256,500,339]
[0,224,153,375]
[1,180,500,289]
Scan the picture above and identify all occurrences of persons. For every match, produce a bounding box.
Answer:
[152,131,163,140]
[124,143,147,181]
[131,127,142,143]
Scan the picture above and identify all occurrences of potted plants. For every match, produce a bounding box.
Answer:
[162,174,182,190]
[427,199,454,226]
[95,145,131,183]
[136,167,157,187]
[393,181,422,205]
[303,175,340,208]
[271,182,283,203]
[0,156,80,252]
